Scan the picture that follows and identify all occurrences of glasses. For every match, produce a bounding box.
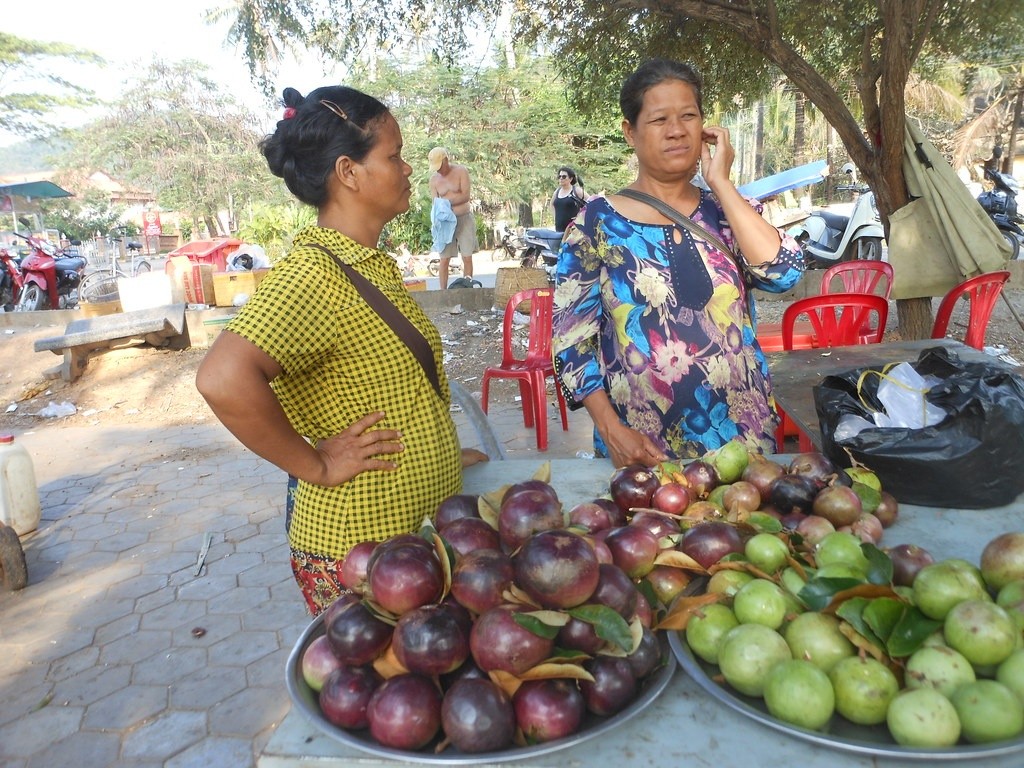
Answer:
[557,174,570,179]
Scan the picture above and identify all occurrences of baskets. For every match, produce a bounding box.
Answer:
[77,268,129,319]
[493,268,549,316]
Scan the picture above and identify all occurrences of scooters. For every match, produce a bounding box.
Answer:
[788,161,886,268]
[960,145,1024,260]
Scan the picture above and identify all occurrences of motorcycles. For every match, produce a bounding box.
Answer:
[518,172,588,283]
[428,254,464,277]
[491,224,527,262]
[0,217,88,311]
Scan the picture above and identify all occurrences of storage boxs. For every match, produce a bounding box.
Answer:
[117,237,268,312]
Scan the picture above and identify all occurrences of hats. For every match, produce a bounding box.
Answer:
[428,147,447,172]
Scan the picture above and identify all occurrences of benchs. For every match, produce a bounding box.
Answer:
[33,302,188,382]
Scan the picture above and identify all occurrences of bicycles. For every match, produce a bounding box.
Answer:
[76,225,152,302]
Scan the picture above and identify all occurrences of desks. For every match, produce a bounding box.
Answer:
[757,323,816,437]
[254,338,1024,768]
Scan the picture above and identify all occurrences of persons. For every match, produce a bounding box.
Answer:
[196,86,488,618]
[427,146,479,290]
[551,61,805,471]
[551,167,590,232]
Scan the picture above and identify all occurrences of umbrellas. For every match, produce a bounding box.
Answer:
[889,108,1024,331]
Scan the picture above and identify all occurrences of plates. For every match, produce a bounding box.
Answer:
[661,624,1024,760]
[282,603,676,766]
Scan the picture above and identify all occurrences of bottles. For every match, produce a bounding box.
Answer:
[0,435,41,536]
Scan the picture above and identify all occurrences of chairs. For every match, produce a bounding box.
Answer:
[774,293,890,453]
[481,288,568,450]
[931,271,1010,350]
[821,260,894,325]
